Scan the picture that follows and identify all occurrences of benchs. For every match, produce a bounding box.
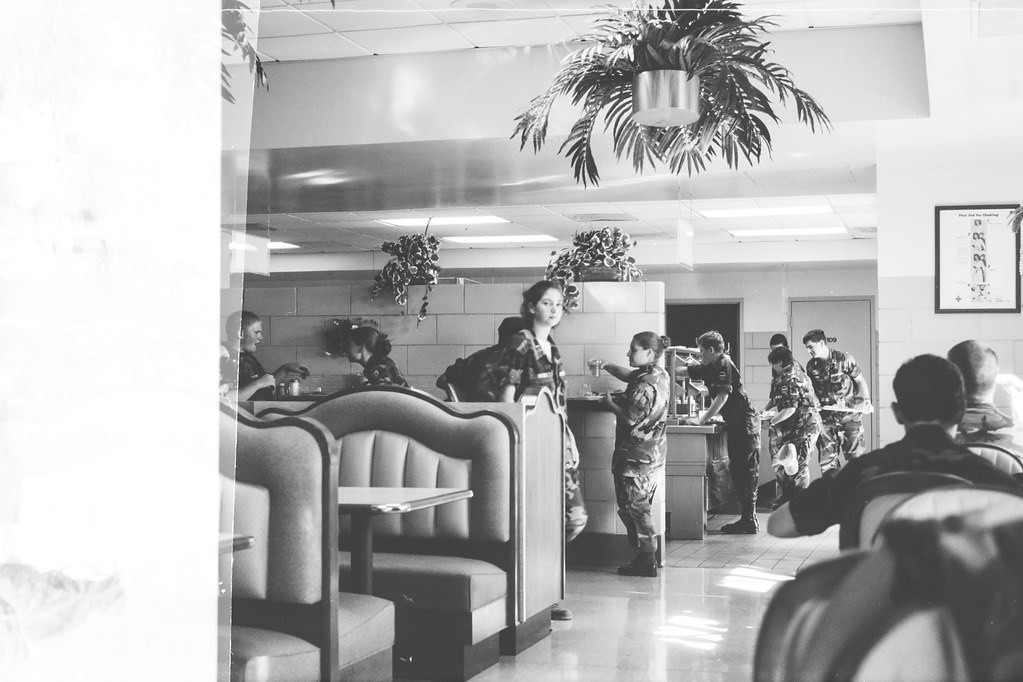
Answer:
[217,391,517,682]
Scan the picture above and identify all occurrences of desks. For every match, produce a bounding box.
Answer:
[336,485,473,595]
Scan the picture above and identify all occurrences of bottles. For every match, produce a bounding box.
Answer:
[289,379,300,396]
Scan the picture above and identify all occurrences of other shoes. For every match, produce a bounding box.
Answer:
[726,519,759,534]
[618,557,658,576]
[552,607,573,620]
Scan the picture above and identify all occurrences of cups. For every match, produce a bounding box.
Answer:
[579,384,591,396]
[592,361,601,378]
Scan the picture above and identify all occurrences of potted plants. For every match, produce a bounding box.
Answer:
[509,0,834,189]
[368,217,441,330]
[545,224,641,316]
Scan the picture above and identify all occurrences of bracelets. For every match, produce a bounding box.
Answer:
[362,381,372,386]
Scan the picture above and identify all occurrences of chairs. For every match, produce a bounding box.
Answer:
[760,442,1023,682]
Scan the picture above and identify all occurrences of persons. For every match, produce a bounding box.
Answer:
[343,326,410,390]
[587,331,671,579]
[759,329,1021,553]
[970,218,987,301]
[667,329,760,534]
[498,281,589,620]
[220,310,311,401]
